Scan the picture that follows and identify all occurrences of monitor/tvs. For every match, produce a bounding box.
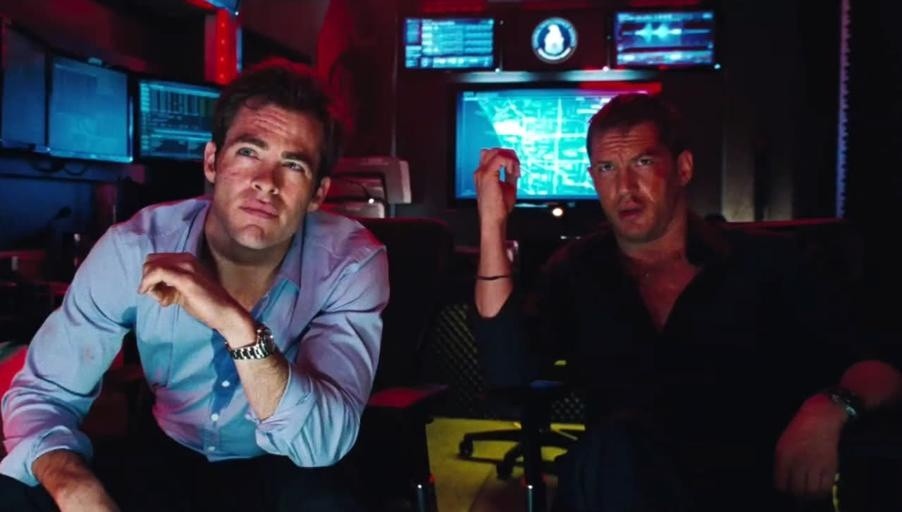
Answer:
[447,89,648,199]
[403,15,502,73]
[47,48,136,163]
[610,8,720,68]
[138,74,221,160]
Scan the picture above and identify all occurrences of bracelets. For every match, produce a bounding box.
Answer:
[474,273,512,282]
[823,381,865,436]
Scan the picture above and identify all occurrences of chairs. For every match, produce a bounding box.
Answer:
[451,238,590,491]
[717,214,848,395]
[348,214,452,512]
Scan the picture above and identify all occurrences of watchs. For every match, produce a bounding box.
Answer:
[224,323,279,362]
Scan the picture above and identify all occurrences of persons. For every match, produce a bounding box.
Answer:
[0,65,397,512]
[471,87,902,511]
